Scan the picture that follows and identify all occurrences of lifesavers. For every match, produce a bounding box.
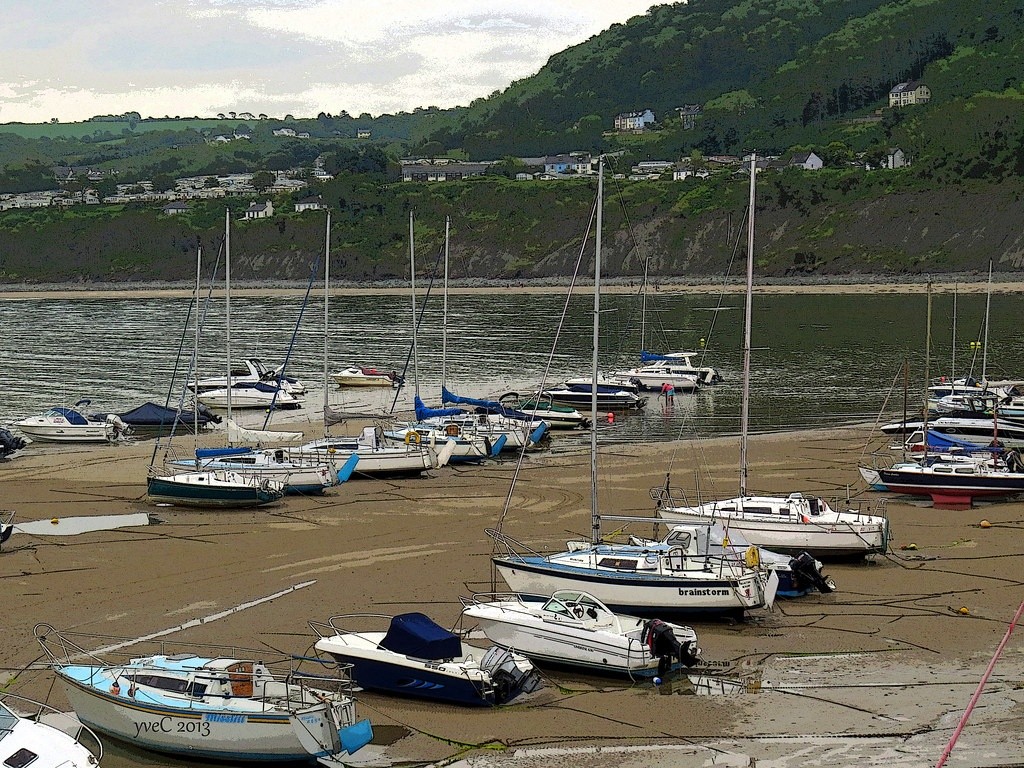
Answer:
[404,432,421,446]
[745,545,760,569]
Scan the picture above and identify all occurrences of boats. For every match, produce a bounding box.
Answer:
[12,380,136,444]
[186,357,310,395]
[0,509,18,544]
[33,621,375,762]
[0,427,28,460]
[306,611,547,706]
[88,394,223,430]
[329,362,407,388]
[457,588,702,677]
[0,690,105,768]
[561,500,837,598]
[192,370,306,409]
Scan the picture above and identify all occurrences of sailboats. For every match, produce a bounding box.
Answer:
[480,153,781,623]
[851,256,1024,507]
[648,147,892,560]
[142,203,724,507]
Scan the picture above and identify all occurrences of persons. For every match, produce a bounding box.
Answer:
[659,383,674,401]
